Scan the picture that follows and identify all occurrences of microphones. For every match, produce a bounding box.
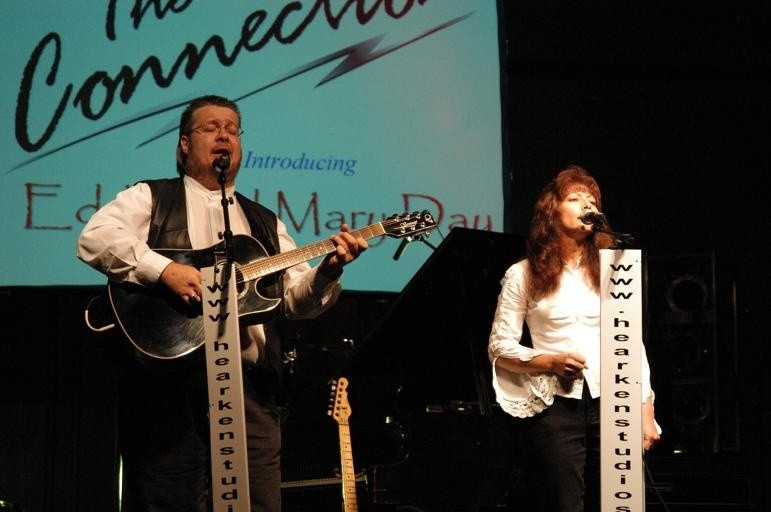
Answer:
[581,210,604,224]
[212,154,231,176]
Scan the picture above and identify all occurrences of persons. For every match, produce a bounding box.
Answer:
[487,162,663,512]
[70,94,375,512]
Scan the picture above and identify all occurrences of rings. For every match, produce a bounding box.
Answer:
[191,292,198,301]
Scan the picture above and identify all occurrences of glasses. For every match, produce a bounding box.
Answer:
[183,118,244,138]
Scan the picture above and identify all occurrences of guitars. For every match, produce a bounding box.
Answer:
[327,377,357,511]
[109,209,437,360]
